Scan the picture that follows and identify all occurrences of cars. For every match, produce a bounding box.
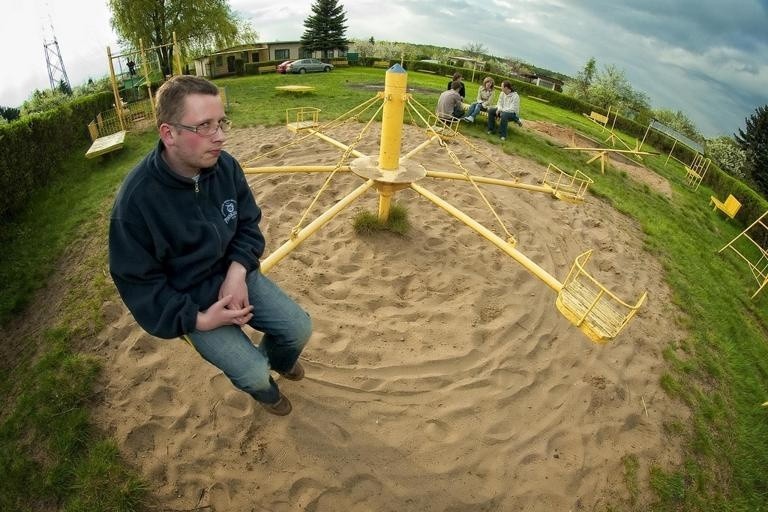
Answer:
[276,58,334,74]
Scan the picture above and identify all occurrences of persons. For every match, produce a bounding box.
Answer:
[462,77,495,123]
[486,80,521,142]
[447,72,465,102]
[109,74,312,417]
[434,81,465,127]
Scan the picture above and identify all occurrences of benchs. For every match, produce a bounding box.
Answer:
[708,194,742,221]
[460,101,501,122]
[590,111,609,125]
[275,85,315,94]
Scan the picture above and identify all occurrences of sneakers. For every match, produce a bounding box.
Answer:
[259,392,292,416]
[280,360,305,382]
[462,116,474,124]
[488,130,492,135]
[501,137,505,140]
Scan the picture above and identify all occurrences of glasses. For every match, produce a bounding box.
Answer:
[168,117,231,137]
